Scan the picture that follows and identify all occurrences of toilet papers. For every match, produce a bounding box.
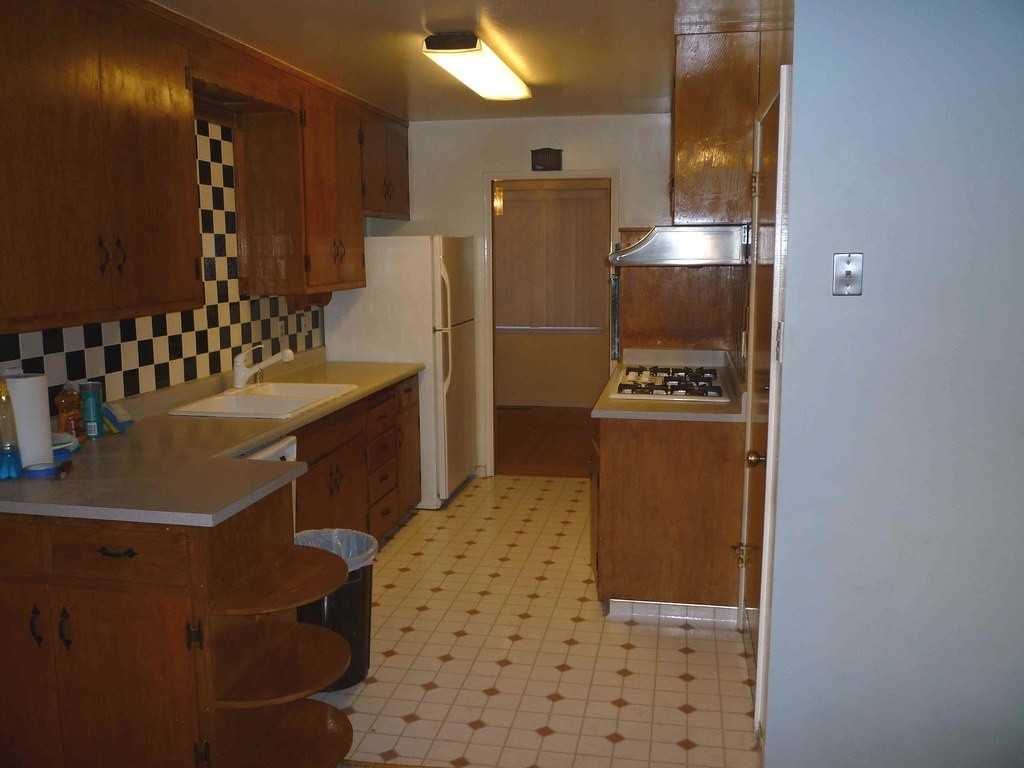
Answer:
[4,373,54,468]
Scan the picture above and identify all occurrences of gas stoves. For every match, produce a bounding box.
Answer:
[609,365,730,403]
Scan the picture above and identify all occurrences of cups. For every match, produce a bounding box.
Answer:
[5,372,54,469]
[79,382,103,438]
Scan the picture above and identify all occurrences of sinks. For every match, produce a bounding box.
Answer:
[215,382,358,395]
[168,396,341,419]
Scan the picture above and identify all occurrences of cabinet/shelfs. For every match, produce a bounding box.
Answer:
[0,0,207,335]
[362,101,410,222]
[0,348,427,768]
[233,75,367,296]
[670,32,761,225]
[590,362,748,621]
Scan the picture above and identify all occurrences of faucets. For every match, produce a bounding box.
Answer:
[233,344,294,388]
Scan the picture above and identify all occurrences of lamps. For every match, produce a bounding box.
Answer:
[422,30,533,102]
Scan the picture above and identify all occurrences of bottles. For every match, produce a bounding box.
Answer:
[0,377,23,481]
[54,384,88,443]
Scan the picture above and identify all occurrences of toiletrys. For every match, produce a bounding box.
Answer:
[56,380,86,443]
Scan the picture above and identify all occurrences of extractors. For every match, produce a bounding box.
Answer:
[608,224,748,267]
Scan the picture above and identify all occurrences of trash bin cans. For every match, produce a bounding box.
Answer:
[295,527,379,690]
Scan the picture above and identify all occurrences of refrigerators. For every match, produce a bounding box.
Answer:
[323,235,478,510]
